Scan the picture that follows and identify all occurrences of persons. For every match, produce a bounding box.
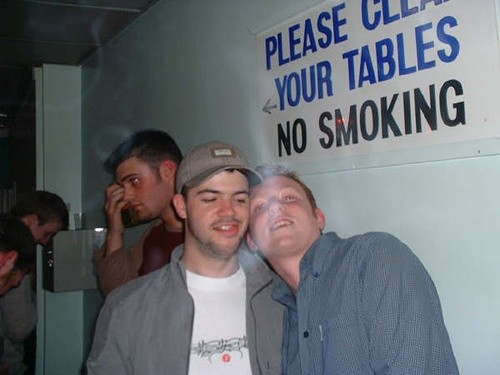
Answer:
[96,130,185,297]
[86,142,286,375]
[246,164,459,375]
[0,191,68,375]
[0,213,36,298]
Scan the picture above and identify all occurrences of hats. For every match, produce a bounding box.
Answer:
[174,140,264,194]
